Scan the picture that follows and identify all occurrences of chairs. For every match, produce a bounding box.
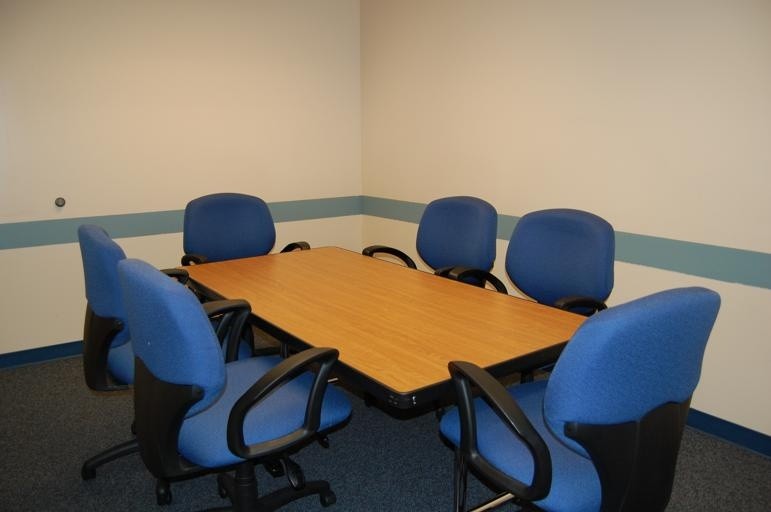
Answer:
[358,195,501,291]
[67,257,356,505]
[70,223,257,484]
[432,285,724,503]
[180,189,314,264]
[447,205,619,386]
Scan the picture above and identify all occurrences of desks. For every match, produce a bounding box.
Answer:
[172,242,602,424]
